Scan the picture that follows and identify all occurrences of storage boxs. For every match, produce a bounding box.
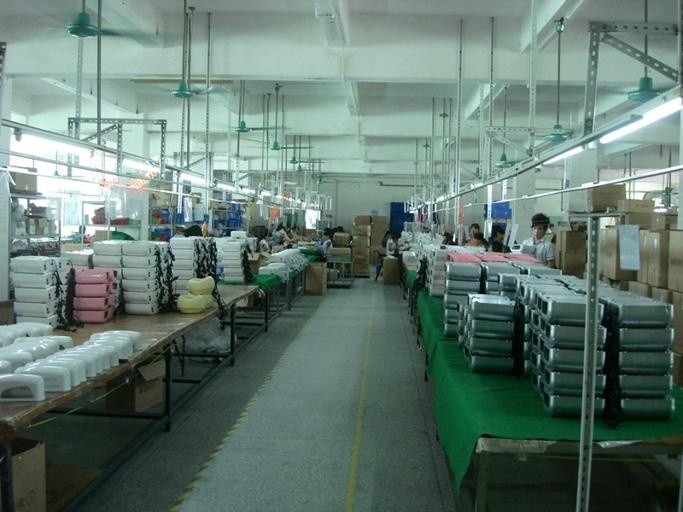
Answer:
[553,184,683,384]
[325,212,400,289]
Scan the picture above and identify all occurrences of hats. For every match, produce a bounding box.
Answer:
[531,213,549,227]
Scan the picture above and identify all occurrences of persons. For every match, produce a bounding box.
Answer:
[521,213,558,268]
[547,223,555,233]
[385,231,404,258]
[70,225,87,240]
[256,221,307,254]
[381,230,392,248]
[442,222,506,252]
[318,225,353,271]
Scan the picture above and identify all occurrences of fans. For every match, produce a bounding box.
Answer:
[616,0,667,104]
[232,79,284,153]
[531,17,583,145]
[488,83,519,170]
[52,1,204,99]
